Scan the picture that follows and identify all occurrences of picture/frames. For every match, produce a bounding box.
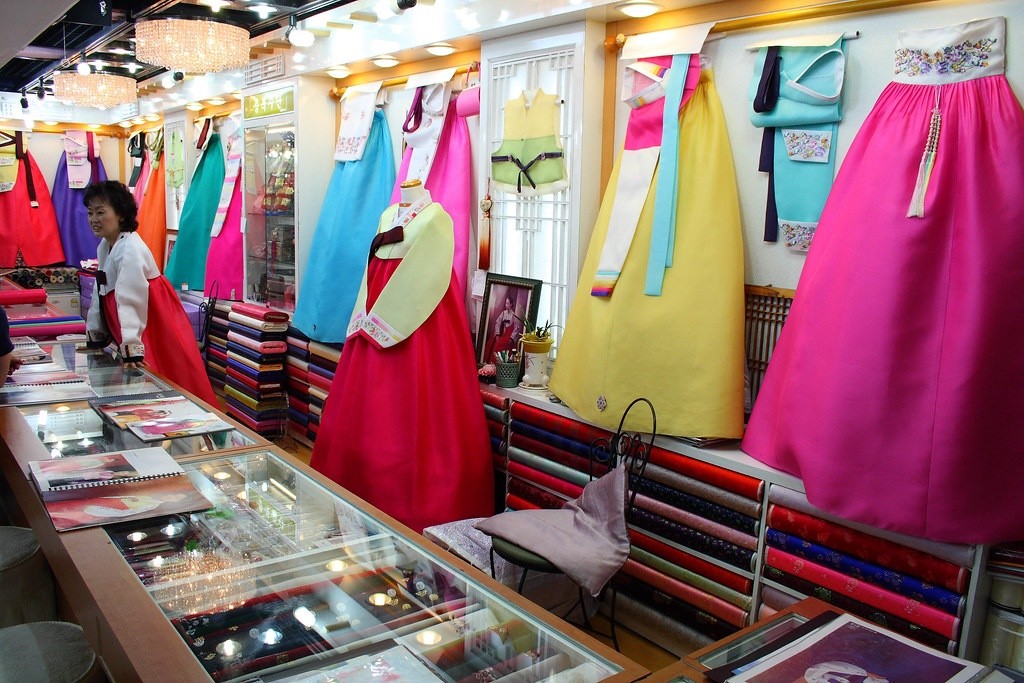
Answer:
[474,272,544,386]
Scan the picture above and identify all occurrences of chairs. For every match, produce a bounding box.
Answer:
[197,279,219,368]
[482,392,660,656]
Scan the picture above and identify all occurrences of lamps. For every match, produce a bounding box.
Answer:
[284,0,460,78]
[197,0,232,12]
[615,3,661,20]
[245,3,277,18]
[18,16,251,130]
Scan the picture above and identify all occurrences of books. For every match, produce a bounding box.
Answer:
[27,446,212,532]
[87,390,236,442]
[0,336,97,407]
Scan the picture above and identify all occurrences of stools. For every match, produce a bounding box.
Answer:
[0,524,59,621]
[0,622,95,683]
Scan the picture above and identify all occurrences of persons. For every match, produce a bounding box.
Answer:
[0,306,24,387]
[483,296,520,364]
[77,180,222,411]
[308,178,495,534]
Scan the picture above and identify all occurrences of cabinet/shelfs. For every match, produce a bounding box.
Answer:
[236,125,302,321]
